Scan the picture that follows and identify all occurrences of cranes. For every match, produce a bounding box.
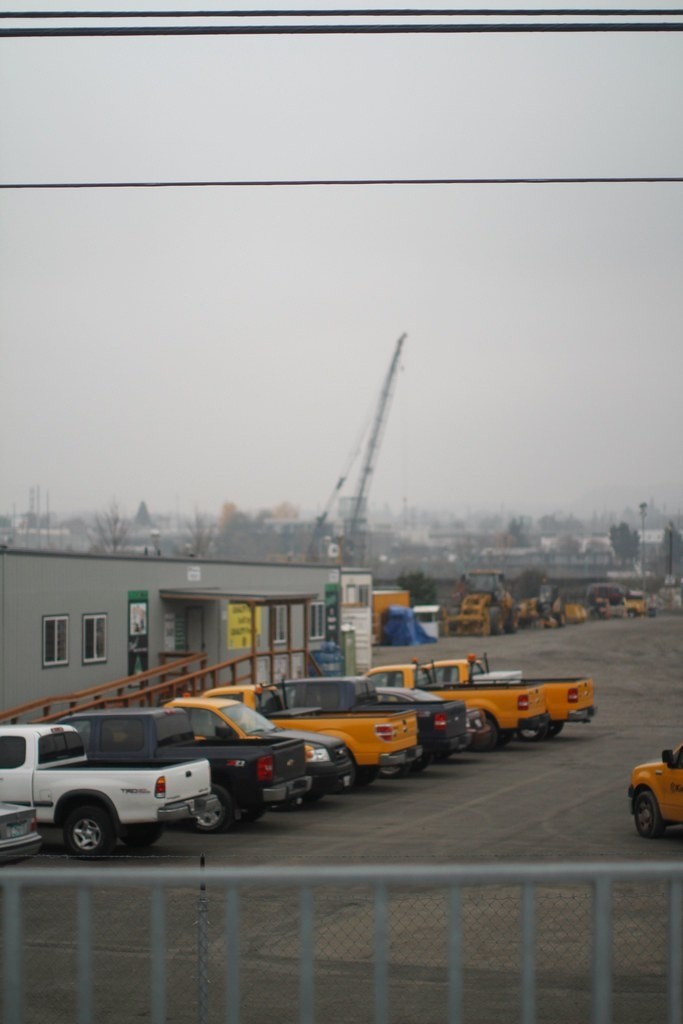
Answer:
[304,326,408,567]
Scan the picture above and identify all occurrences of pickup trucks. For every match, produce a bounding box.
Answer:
[362,657,548,757]
[198,671,426,792]
[0,723,215,861]
[422,653,599,747]
[44,705,312,834]
[266,675,466,782]
[110,693,356,806]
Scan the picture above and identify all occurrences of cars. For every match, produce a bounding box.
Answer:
[0,797,44,867]
[627,739,682,840]
[372,685,500,774]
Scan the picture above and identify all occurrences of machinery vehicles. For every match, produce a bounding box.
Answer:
[441,569,647,640]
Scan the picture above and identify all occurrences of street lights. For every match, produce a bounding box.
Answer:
[638,502,649,575]
